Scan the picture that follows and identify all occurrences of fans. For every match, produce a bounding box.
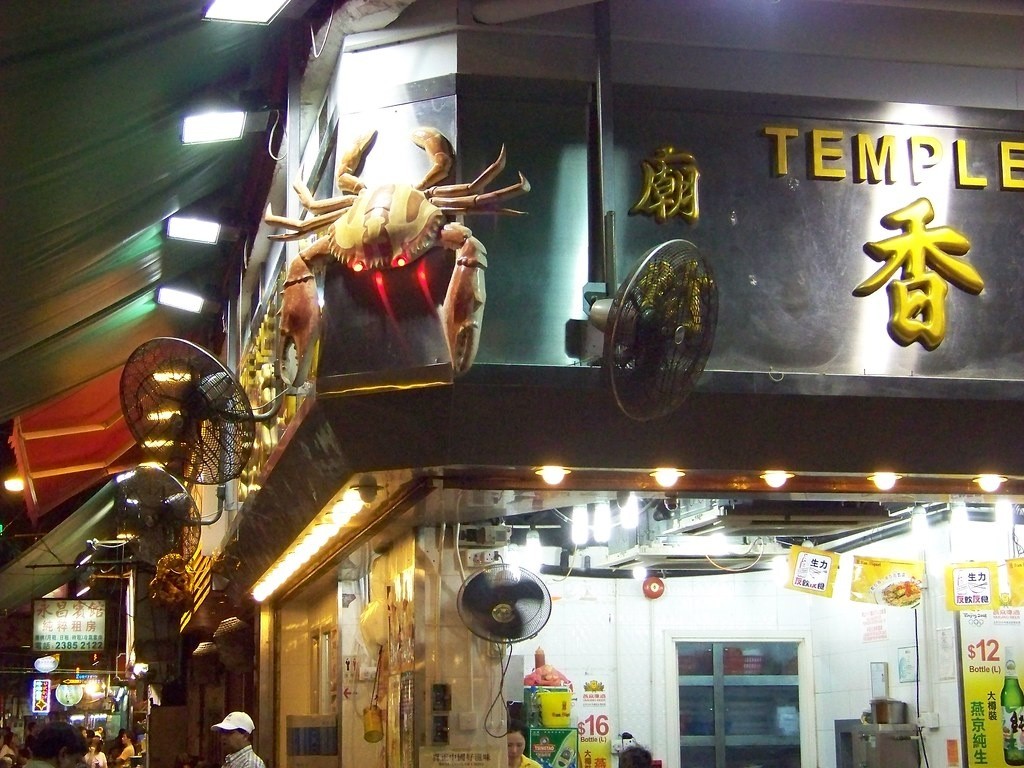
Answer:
[119,336,285,483]
[115,465,226,568]
[457,563,553,658]
[584,240,719,423]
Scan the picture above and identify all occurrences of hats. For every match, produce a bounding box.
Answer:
[211,711,255,735]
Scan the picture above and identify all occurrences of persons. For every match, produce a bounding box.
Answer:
[211,711,265,768]
[0,710,134,768]
[507,719,542,768]
[619,746,652,768]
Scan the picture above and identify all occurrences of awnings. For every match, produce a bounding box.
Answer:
[0,0,319,612]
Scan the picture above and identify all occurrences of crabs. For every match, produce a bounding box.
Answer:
[265,127,532,373]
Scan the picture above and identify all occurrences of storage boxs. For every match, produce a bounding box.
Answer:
[522,685,579,768]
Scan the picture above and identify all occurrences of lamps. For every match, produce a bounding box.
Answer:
[972,475,1009,493]
[761,471,795,489]
[650,469,689,492]
[868,474,903,491]
[166,204,244,245]
[200,0,318,26]
[534,466,572,485]
[156,281,225,314]
[180,102,272,145]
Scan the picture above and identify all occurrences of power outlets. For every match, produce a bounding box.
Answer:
[466,551,494,568]
